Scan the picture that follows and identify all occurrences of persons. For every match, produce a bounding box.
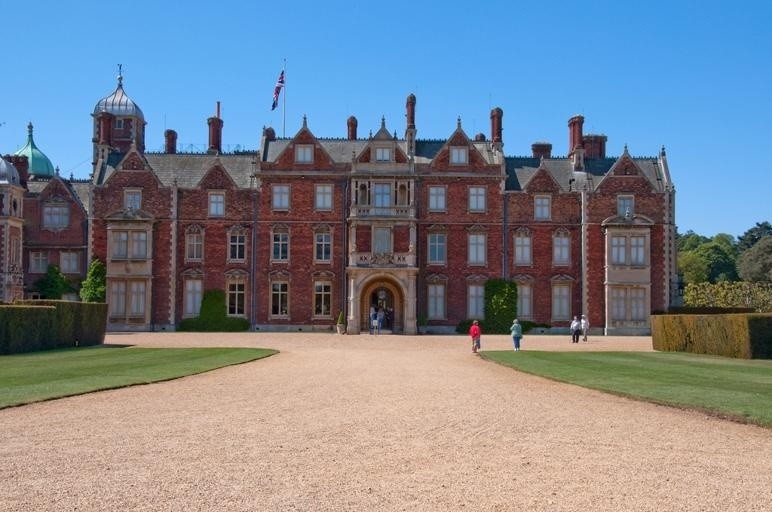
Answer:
[570,315,581,343]
[510,319,522,351]
[469,319,481,353]
[369,306,393,335]
[580,314,590,341]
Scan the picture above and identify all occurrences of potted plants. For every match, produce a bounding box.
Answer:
[336,311,347,335]
[417,310,429,334]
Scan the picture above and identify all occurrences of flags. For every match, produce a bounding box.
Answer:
[272,69,285,110]
